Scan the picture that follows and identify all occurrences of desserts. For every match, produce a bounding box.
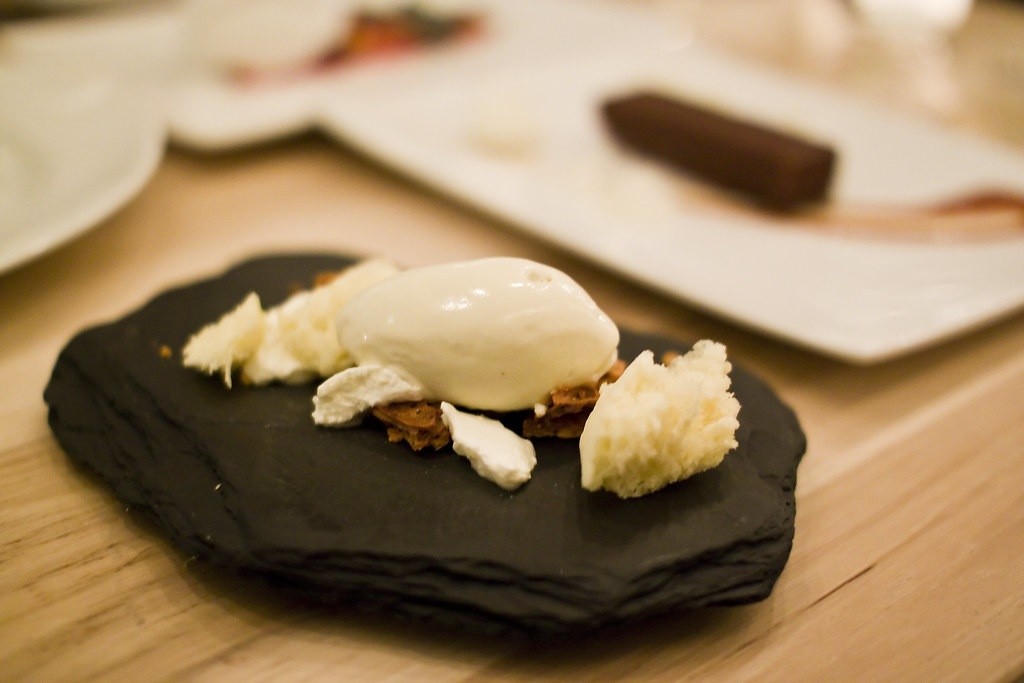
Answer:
[311,252,620,487]
[603,85,834,215]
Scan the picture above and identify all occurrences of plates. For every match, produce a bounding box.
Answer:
[0,1,687,157]
[0,68,168,277]
[317,42,1023,361]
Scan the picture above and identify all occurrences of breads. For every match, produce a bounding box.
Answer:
[580,337,740,498]
[183,261,387,390]
